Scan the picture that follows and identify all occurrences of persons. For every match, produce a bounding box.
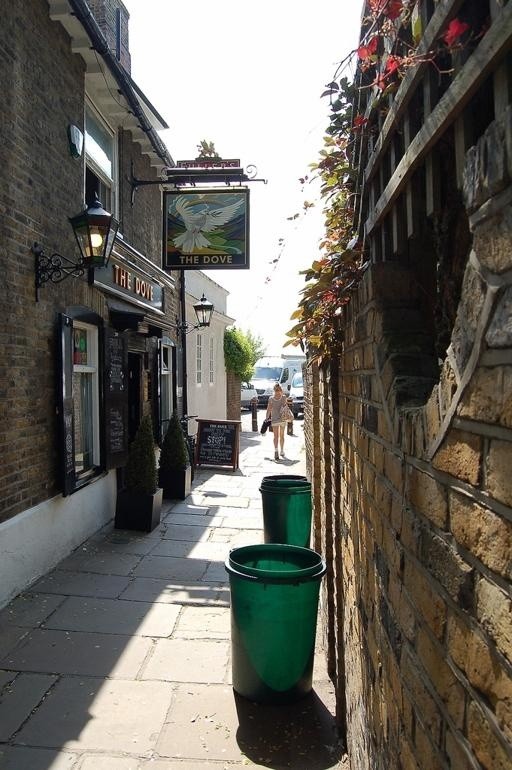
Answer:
[265,384,290,460]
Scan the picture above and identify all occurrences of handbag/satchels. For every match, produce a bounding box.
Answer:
[261,418,270,434]
[279,403,294,424]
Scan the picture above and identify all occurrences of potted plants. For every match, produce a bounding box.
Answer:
[112,413,164,534]
[158,405,194,500]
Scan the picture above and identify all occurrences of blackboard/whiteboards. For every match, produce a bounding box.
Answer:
[197,421,237,465]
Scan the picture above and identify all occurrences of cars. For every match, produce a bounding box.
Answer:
[240,381,260,410]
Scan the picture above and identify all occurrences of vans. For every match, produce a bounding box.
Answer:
[286,372,303,418]
[248,355,305,409]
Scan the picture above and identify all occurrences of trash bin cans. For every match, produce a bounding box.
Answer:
[224,544,328,705]
[259,475,312,548]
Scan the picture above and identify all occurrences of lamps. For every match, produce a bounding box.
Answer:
[29,189,123,305]
[174,293,215,342]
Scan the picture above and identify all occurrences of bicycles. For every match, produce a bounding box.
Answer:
[160,414,199,485]
